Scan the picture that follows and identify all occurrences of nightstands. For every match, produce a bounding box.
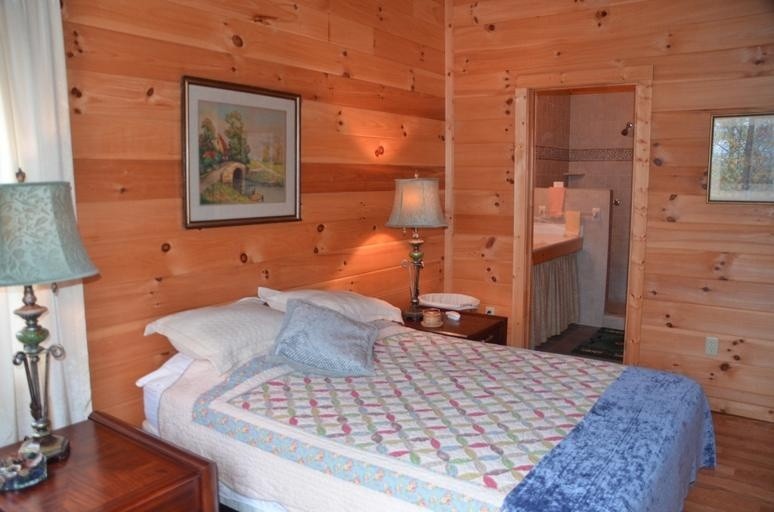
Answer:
[404,307,507,346]
[0,410,219,512]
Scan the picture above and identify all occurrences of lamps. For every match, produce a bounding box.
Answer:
[1,166,100,463]
[384,172,448,320]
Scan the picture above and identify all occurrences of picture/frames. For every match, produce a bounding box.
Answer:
[182,76,301,229]
[706,111,774,205]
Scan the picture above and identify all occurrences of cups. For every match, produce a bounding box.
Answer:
[422,308,441,325]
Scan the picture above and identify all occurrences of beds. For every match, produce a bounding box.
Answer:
[136,321,716,512]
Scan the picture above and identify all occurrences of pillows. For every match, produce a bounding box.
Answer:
[258,287,404,325]
[143,296,285,376]
[266,298,378,377]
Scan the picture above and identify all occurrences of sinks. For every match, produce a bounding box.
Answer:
[533,232,576,249]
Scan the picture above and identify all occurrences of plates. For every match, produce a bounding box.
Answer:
[421,321,443,327]
